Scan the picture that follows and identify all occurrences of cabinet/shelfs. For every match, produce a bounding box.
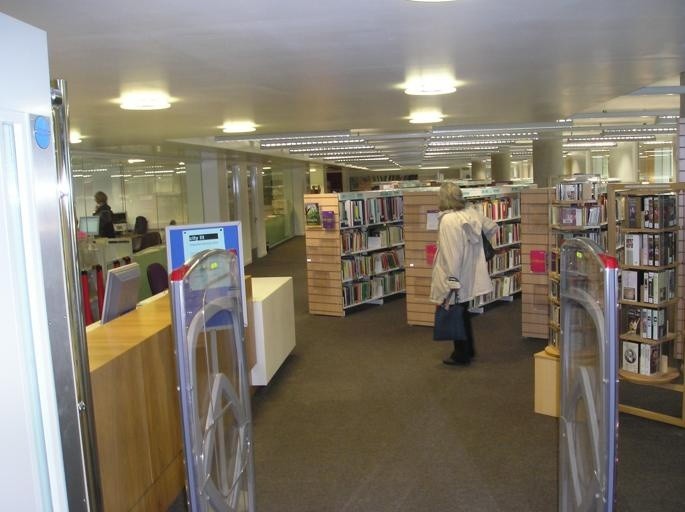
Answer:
[519,185,607,340]
[399,186,521,329]
[605,183,684,386]
[303,187,404,319]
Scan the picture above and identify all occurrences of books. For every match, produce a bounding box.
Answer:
[621,194,674,377]
[339,196,406,307]
[475,198,523,307]
[548,182,610,344]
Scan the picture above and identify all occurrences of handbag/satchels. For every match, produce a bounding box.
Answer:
[433,277,473,342]
[481,226,495,261]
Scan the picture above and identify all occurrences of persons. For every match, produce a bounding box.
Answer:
[93,191,117,238]
[430,182,498,365]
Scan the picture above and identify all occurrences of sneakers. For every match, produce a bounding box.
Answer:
[443,358,464,366]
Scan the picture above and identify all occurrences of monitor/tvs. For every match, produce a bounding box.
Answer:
[100,261,140,324]
[78,216,101,237]
